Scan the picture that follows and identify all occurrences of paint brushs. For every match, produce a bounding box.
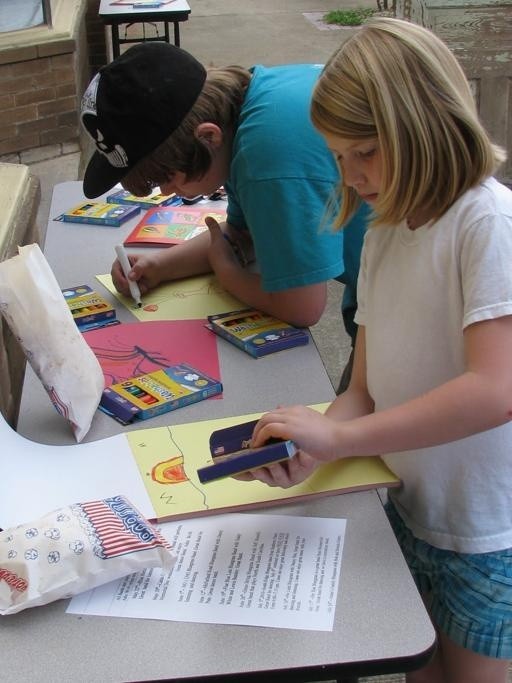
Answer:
[114,244,142,308]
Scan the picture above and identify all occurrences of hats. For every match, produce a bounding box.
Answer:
[81,41,208,200]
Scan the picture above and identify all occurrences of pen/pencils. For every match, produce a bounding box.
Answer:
[71,304,106,314]
[125,385,157,405]
[223,314,270,327]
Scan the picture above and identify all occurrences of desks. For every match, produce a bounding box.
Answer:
[98,0,195,69]
[0,163,445,681]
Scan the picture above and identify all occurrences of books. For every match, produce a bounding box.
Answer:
[109,0,175,5]
[123,206,228,248]
[0,402,402,530]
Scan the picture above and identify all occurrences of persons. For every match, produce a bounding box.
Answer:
[81,42,376,396]
[231,16,512,683]
